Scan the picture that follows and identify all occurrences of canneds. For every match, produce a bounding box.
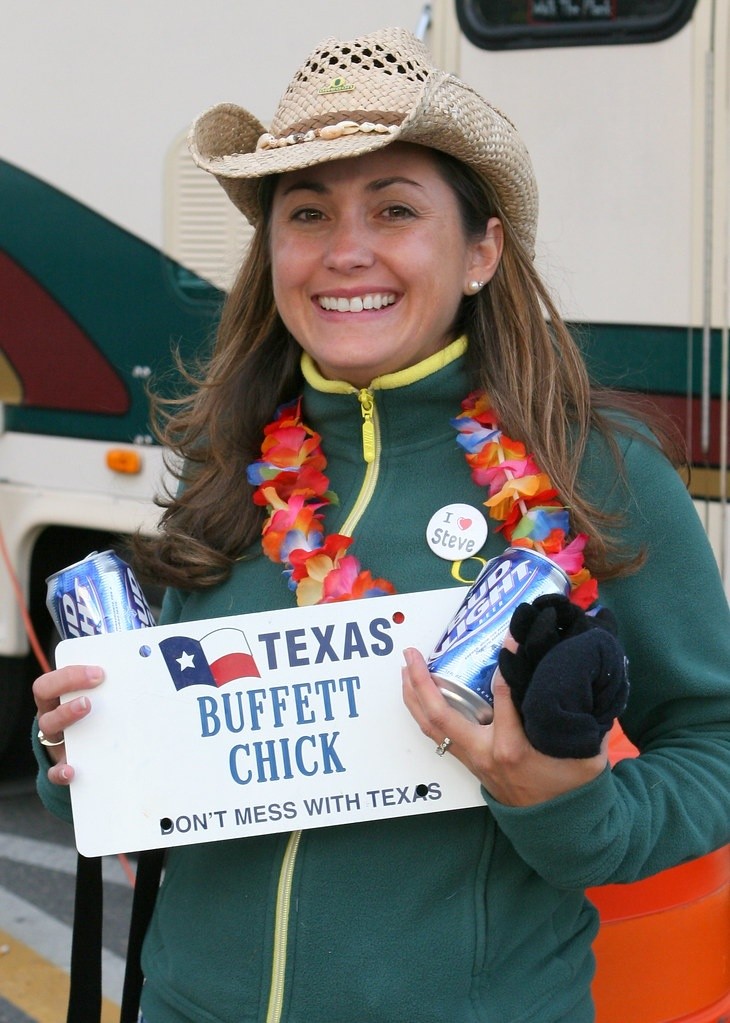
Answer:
[43,550,159,640]
[425,547,572,725]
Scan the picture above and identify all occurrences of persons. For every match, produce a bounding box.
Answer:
[30,29,730,1023]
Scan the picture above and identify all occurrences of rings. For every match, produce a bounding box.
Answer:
[435,736,452,759]
[37,728,66,747]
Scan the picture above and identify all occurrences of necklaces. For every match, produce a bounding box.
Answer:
[244,386,600,618]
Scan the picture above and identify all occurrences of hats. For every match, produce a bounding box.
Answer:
[186,25,538,266]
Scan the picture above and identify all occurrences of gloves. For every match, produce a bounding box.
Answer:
[498,591,629,760]
